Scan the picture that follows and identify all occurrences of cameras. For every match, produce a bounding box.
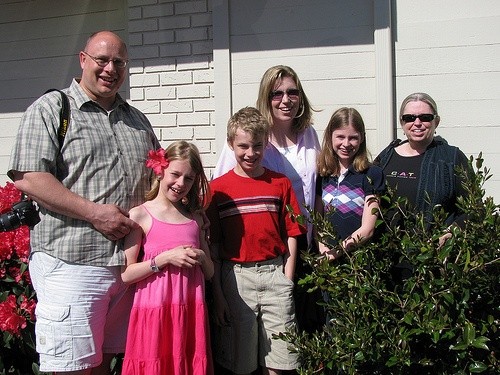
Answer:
[0,198,41,233]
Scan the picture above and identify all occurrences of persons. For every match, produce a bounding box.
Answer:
[7,31,211,375]
[120,141,215,375]
[372,92,478,300]
[214,65,322,251]
[201,107,308,375]
[313,107,384,324]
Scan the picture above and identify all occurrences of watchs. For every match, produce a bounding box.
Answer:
[150,257,160,273]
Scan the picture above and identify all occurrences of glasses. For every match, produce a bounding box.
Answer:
[83,51,127,69]
[401,113,437,123]
[267,89,300,101]
